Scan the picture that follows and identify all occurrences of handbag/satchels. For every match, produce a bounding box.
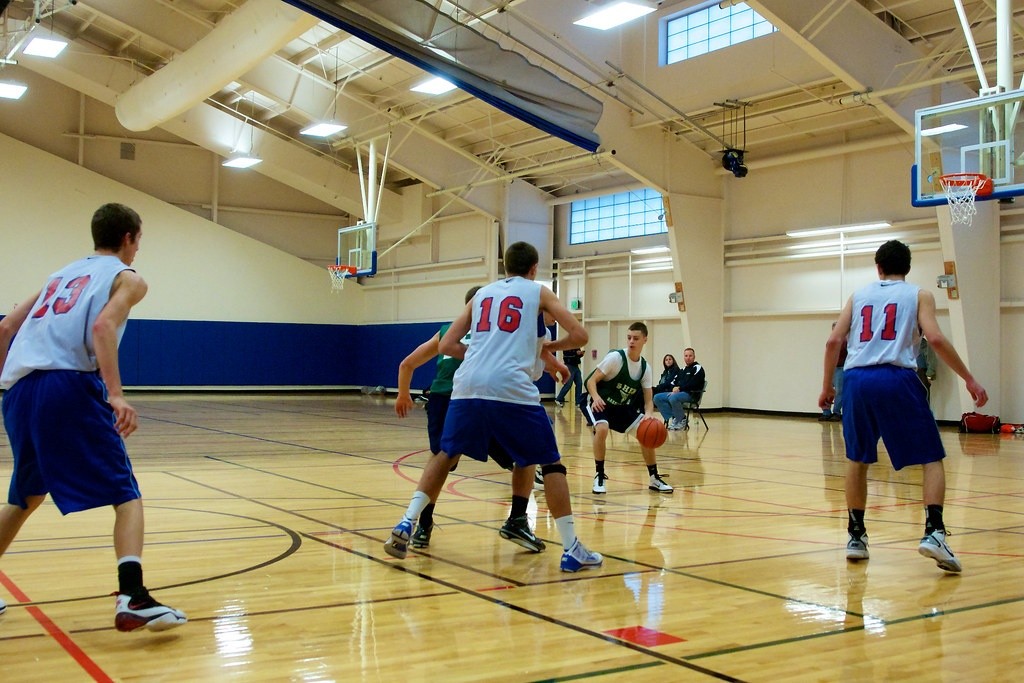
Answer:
[959,412,1000,434]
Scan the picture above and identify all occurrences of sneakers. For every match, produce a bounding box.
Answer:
[671,418,677,430]
[675,418,689,430]
[560,536,603,573]
[649,473,673,492]
[917,529,962,572]
[383,514,418,559]
[532,468,544,484]
[499,513,546,553]
[593,471,609,494]
[847,527,870,558]
[110,586,188,633]
[410,518,434,548]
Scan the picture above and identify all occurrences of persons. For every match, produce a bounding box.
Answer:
[916,332,938,404]
[653,348,705,430]
[818,322,848,422]
[383,242,605,573]
[554,349,585,407]
[818,240,988,573]
[587,321,673,495]
[0,202,188,633]
[579,368,597,426]
[652,354,680,407]
[532,309,556,490]
[395,286,571,553]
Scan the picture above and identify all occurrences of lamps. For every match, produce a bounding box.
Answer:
[221,92,263,169]
[409,0,459,95]
[299,47,349,137]
[0,35,29,99]
[571,0,659,31]
[21,0,68,59]
[718,104,749,178]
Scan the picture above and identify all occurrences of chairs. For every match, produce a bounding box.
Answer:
[663,380,710,431]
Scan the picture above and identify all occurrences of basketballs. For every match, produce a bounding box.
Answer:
[637,418,668,449]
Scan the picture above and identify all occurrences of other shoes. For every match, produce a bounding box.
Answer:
[554,400,564,407]
[818,415,829,421]
[829,414,841,422]
